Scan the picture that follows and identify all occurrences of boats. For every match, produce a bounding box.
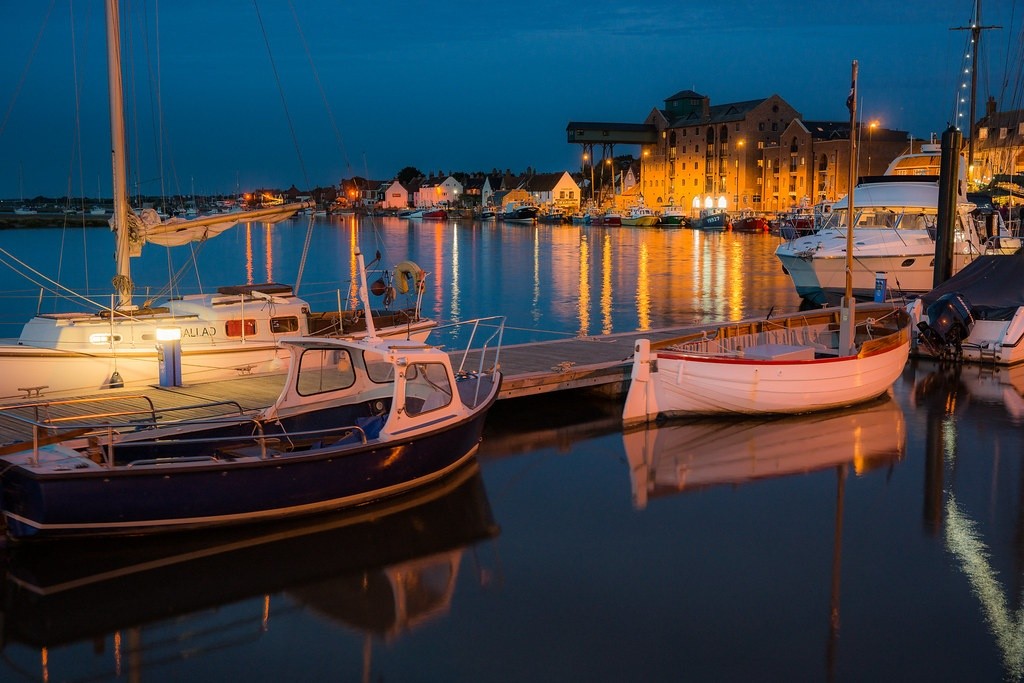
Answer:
[1,454,502,683]
[907,232,1024,367]
[623,385,907,683]
[1,314,506,545]
[906,363,1022,435]
[774,130,1022,303]
[16,198,835,239]
[623,59,914,433]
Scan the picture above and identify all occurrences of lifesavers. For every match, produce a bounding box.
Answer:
[395,260,426,294]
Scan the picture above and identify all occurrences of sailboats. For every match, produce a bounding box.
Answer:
[1,1,437,411]
